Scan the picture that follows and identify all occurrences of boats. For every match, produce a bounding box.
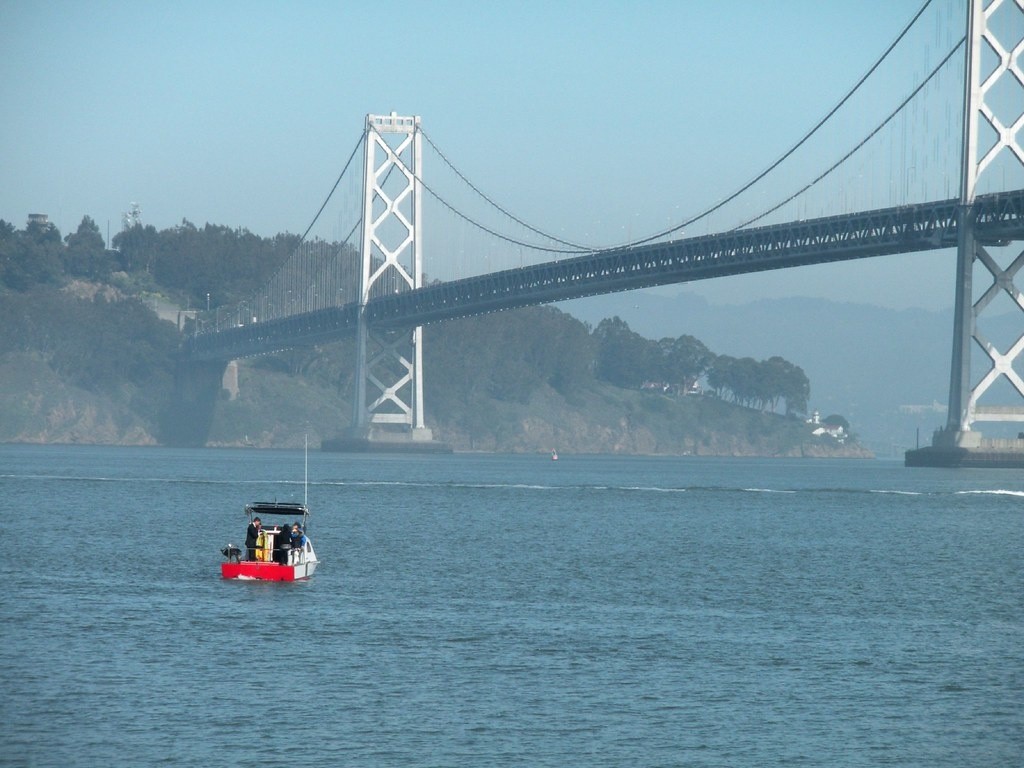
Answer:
[219,502,321,582]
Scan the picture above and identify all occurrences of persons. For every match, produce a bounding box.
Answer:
[274,524,291,565]
[290,522,306,552]
[255,529,269,562]
[244,517,261,562]
